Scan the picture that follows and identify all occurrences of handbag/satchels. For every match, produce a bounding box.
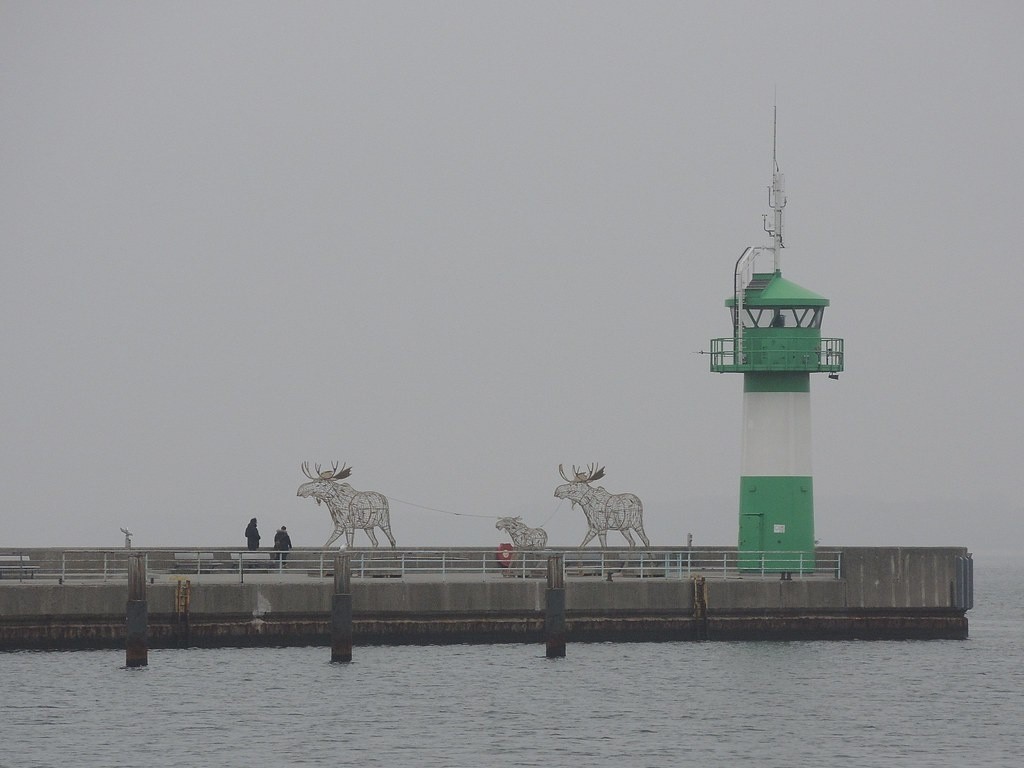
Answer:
[274,541,280,550]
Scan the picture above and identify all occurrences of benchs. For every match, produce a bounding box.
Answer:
[174,553,225,574]
[231,553,276,574]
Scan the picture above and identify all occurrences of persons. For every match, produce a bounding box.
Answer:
[274,526,293,568]
[245,518,261,568]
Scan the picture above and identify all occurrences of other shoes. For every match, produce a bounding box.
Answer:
[248,564,255,568]
[282,563,287,568]
